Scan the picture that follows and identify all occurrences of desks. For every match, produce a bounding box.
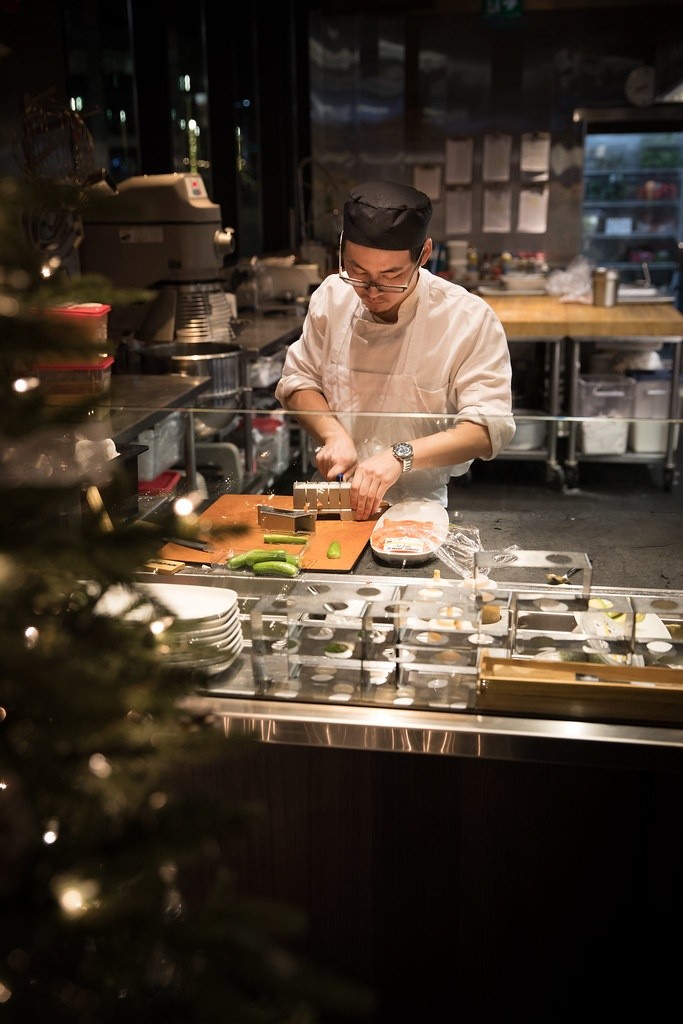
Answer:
[560,295,683,467]
[473,294,568,468]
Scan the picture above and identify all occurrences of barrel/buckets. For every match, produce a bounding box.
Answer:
[149,344,242,412]
[510,408,546,452]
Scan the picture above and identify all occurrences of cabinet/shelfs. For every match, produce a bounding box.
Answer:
[0,375,211,570]
[581,167,683,274]
[232,315,309,495]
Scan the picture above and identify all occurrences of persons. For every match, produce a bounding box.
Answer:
[275,181,516,520]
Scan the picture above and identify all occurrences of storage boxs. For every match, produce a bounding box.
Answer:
[508,409,547,450]
[576,355,683,457]
[15,302,116,407]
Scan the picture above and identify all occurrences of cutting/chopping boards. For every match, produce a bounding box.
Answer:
[158,495,389,571]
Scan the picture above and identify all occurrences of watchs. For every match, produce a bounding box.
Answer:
[391,443,414,474]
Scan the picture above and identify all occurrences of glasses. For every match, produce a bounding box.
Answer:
[338,231,426,293]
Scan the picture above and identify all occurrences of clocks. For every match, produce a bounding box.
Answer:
[623,66,656,109]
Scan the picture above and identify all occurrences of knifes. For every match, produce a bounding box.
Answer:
[337,473,343,492]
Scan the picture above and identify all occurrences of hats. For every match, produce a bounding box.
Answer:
[344,180,433,250]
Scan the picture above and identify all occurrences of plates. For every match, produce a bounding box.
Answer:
[500,274,544,289]
[477,285,547,297]
[98,583,245,673]
[370,502,448,564]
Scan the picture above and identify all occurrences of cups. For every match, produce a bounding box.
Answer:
[592,267,618,307]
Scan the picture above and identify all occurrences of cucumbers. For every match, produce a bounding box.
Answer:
[227,549,300,577]
[326,541,340,559]
[263,534,308,544]
